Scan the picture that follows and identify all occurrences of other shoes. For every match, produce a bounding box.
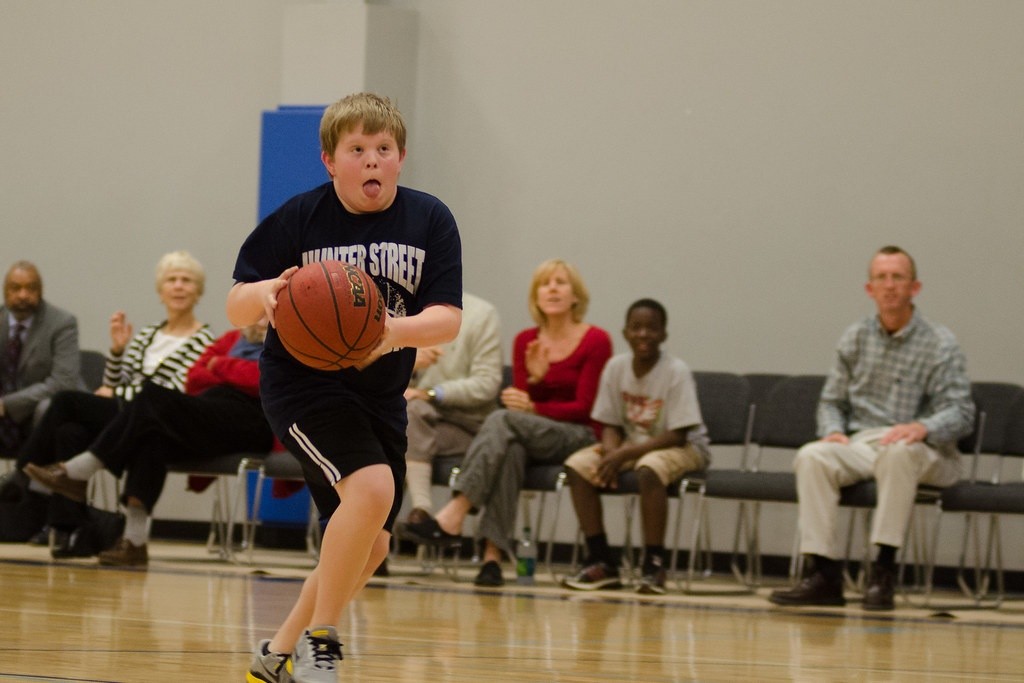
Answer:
[473,560,502,586]
[406,508,463,548]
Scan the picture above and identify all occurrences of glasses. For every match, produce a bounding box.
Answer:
[869,271,914,282]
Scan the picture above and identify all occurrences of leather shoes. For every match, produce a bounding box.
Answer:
[99,540,148,567]
[767,571,844,605]
[861,566,902,609]
[23,462,88,503]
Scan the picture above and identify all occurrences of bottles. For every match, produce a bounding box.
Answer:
[516,526,537,584]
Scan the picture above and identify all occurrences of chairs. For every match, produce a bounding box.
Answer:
[7,345,1024,621]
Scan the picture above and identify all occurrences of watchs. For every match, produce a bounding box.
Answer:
[425,387,436,402]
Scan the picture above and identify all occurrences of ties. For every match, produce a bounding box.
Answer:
[7,324,27,379]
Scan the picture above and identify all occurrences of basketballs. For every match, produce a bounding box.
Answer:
[274,260,386,370]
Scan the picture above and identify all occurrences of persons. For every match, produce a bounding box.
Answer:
[228,94,463,683]
[1,250,305,570]
[769,246,976,610]
[403,259,713,594]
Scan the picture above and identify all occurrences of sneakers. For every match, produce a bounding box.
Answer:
[293,625,343,683]
[631,563,667,595]
[246,639,295,683]
[561,563,623,590]
